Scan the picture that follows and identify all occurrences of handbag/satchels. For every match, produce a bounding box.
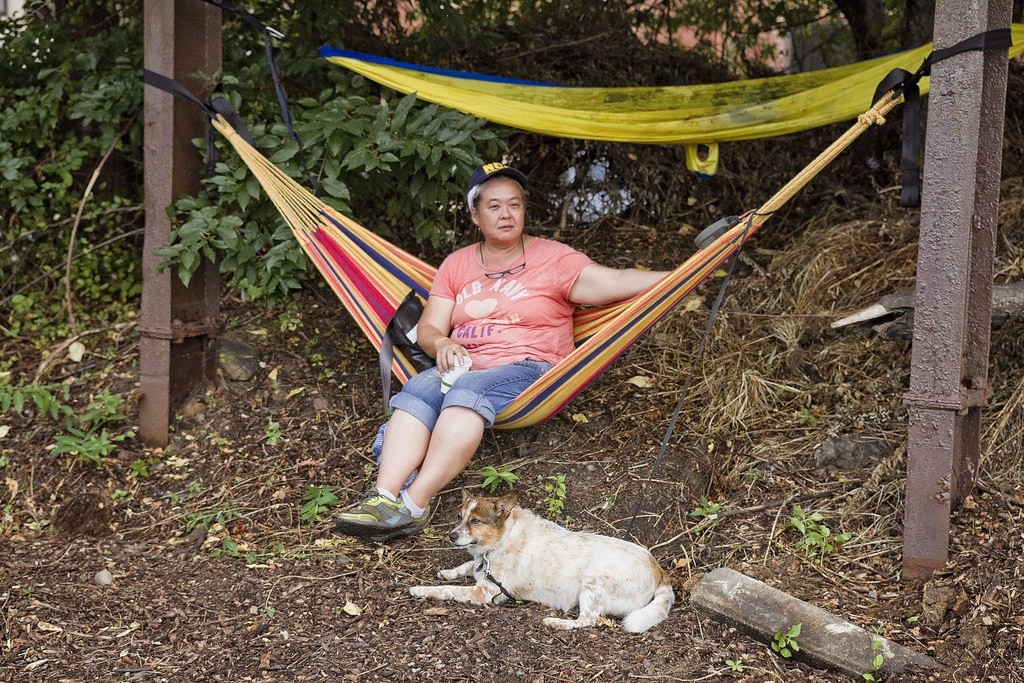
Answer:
[392,293,435,372]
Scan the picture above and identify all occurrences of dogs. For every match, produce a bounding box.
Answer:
[409,485,676,636]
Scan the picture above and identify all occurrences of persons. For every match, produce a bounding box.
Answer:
[337,163,675,541]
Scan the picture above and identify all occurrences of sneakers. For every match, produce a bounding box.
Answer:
[336,491,431,542]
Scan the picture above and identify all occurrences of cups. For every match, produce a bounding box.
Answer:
[441,355,473,394]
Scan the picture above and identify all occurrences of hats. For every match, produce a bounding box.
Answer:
[468,162,529,193]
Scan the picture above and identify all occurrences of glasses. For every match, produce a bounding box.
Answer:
[480,235,526,279]
